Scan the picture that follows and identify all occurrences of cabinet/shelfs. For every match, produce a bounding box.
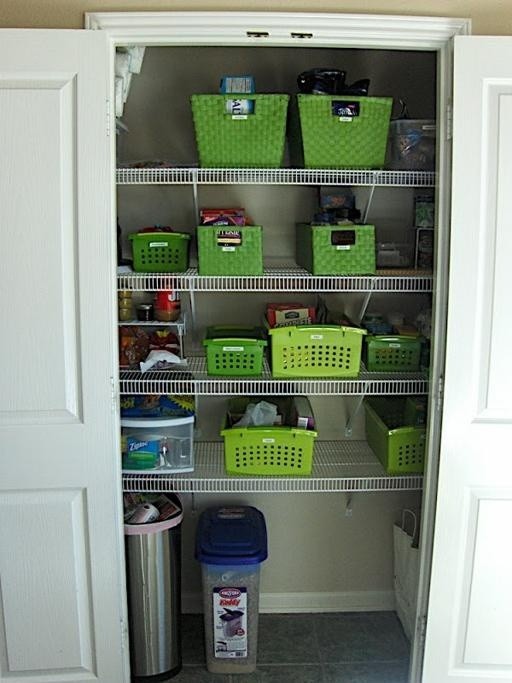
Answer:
[85,10,470,683]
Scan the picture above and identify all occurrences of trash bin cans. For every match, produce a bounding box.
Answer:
[124,474,184,683]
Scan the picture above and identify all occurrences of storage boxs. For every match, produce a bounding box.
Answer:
[384,118,436,171]
[121,416,194,473]
[194,506,268,675]
